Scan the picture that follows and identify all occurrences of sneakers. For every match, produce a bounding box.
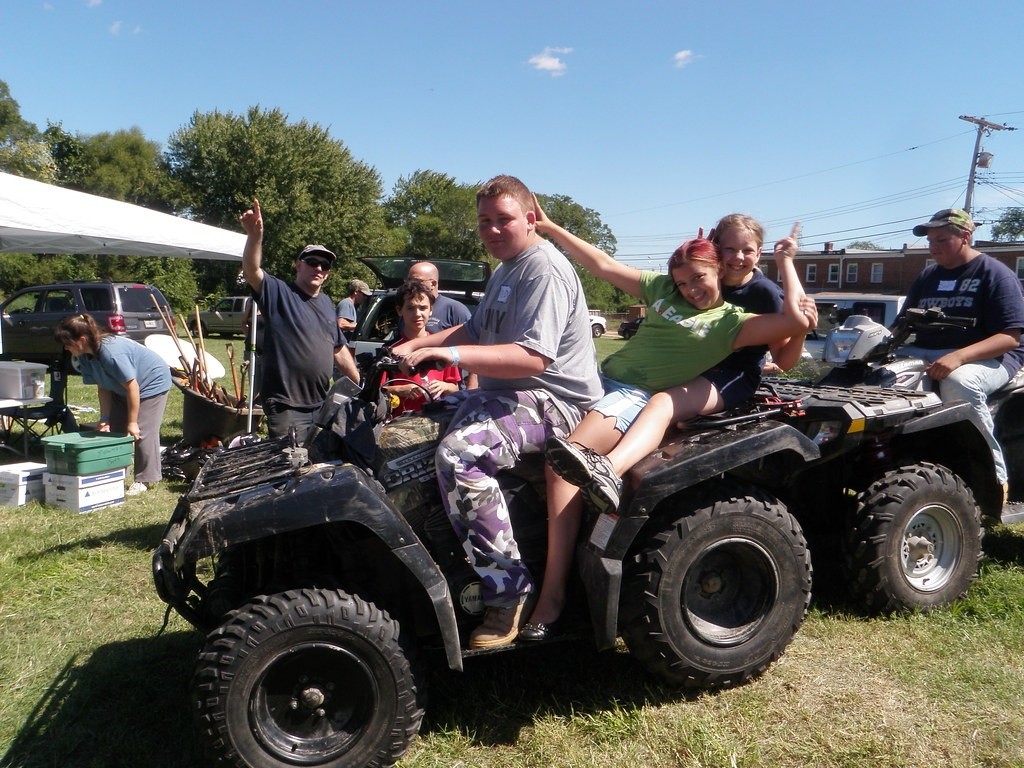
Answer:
[544,435,623,514]
[468,590,534,650]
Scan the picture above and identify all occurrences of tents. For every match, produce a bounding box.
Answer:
[0,172,258,434]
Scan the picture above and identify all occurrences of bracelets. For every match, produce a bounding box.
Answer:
[100,416,110,420]
[449,345,458,366]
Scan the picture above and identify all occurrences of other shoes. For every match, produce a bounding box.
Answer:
[126,481,149,495]
[1001,482,1009,506]
[520,619,558,641]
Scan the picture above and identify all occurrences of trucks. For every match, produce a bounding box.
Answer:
[802,292,915,361]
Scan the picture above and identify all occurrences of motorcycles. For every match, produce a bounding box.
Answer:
[151,342,822,768]
[734,304,1024,621]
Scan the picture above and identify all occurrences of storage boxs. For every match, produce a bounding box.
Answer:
[41,430,135,476]
[0,461,47,507]
[43,467,126,514]
[0,361,49,400]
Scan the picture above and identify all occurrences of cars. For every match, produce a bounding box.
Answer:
[589,316,607,339]
[618,317,648,341]
[342,252,490,367]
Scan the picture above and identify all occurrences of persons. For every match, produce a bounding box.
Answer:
[408,262,478,389]
[239,200,359,437]
[337,281,372,330]
[518,192,810,640]
[380,283,461,419]
[544,214,818,515]
[889,209,1024,504]
[386,176,606,648]
[55,313,172,495]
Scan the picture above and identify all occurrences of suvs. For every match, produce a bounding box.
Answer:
[0,279,181,378]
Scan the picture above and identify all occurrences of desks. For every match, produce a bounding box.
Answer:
[0,396,54,457]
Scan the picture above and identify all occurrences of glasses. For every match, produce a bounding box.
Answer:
[299,257,331,271]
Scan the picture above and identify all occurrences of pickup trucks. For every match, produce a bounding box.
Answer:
[186,296,266,339]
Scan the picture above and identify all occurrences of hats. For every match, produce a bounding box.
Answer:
[350,279,372,296]
[913,207,975,236]
[298,244,337,262]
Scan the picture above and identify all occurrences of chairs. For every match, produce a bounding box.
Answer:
[0,353,70,449]
